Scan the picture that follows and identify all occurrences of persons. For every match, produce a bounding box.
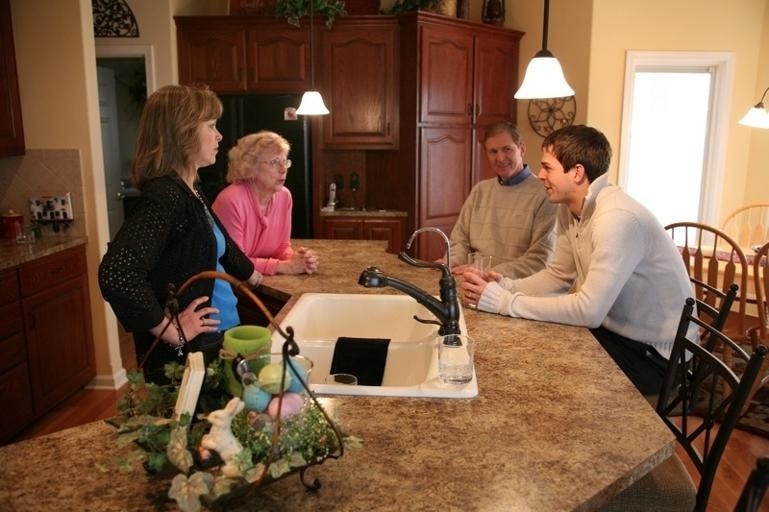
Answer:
[98,84,264,419]
[210,129,320,276]
[460,124,701,397]
[433,122,559,280]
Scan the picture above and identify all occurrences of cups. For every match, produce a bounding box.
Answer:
[223,324,272,395]
[467,252,492,275]
[4,209,25,242]
[235,352,313,431]
[437,334,476,383]
[12,221,36,244]
[323,373,358,385]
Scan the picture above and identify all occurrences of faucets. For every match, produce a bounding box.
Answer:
[358,249,461,346]
[405,227,451,275]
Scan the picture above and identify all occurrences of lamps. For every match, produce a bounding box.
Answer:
[739,81,769,132]
[509,1,579,100]
[293,0,329,117]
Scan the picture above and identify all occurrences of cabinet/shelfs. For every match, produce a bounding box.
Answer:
[173,12,310,97]
[0,0,28,159]
[22,241,98,427]
[407,8,525,125]
[366,124,502,263]
[1,265,33,447]
[311,11,408,152]
[314,211,411,254]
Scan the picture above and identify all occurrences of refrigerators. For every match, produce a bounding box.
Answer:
[194,90,312,240]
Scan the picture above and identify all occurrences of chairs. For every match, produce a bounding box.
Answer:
[643,199,769,512]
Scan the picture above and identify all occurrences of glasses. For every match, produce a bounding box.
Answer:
[257,157,293,170]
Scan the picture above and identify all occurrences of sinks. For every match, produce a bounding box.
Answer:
[268,291,442,389]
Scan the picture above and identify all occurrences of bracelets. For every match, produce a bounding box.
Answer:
[169,323,185,357]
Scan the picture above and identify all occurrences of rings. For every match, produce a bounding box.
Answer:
[468,292,472,298]
[202,317,205,326]
